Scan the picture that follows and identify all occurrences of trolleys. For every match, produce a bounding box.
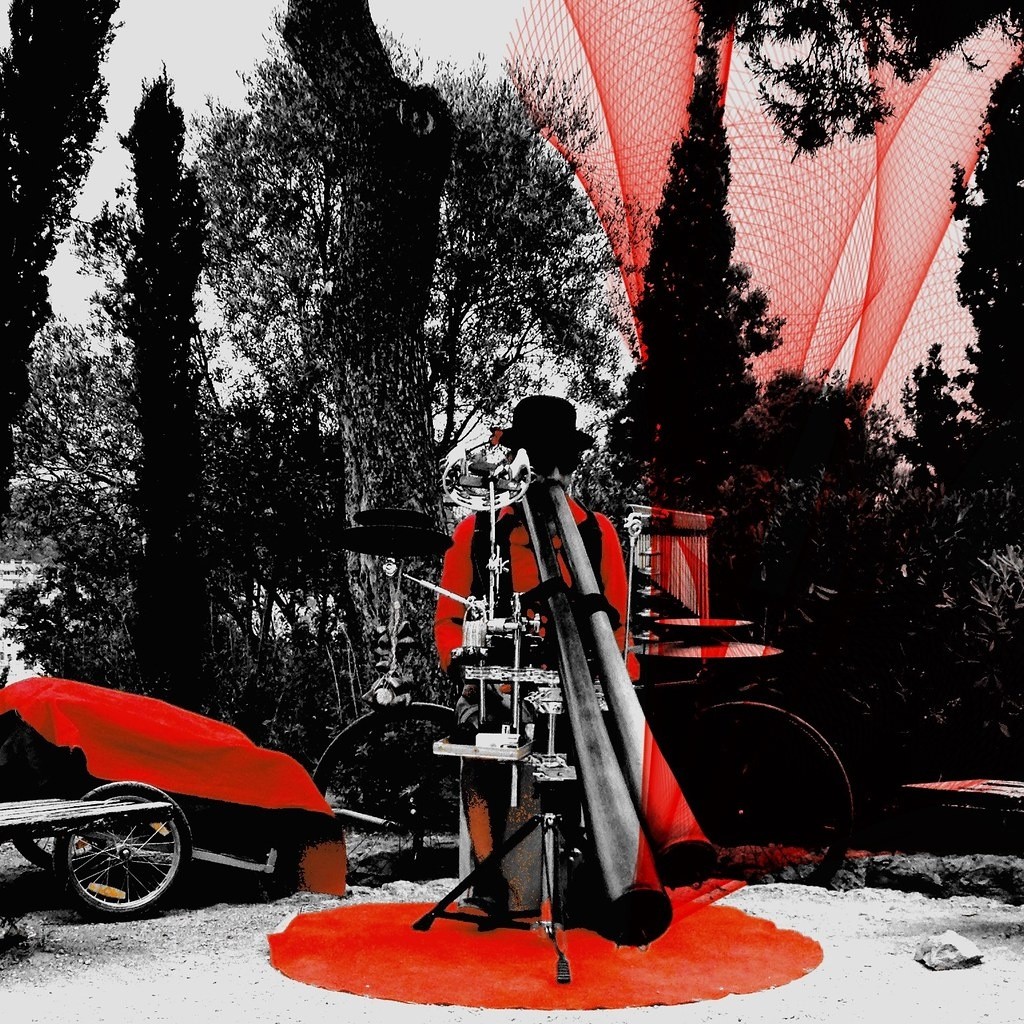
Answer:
[0,676,335,921]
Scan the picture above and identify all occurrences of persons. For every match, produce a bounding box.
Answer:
[433,393,643,918]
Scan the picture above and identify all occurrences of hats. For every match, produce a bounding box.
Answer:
[529,455,582,476]
[492,395,596,452]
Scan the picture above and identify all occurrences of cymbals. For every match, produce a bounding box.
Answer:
[654,614,754,637]
[329,525,455,556]
[625,640,786,659]
[353,507,435,527]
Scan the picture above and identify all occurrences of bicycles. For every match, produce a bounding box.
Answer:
[310,621,854,895]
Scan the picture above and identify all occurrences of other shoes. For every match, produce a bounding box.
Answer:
[458,897,511,922]
